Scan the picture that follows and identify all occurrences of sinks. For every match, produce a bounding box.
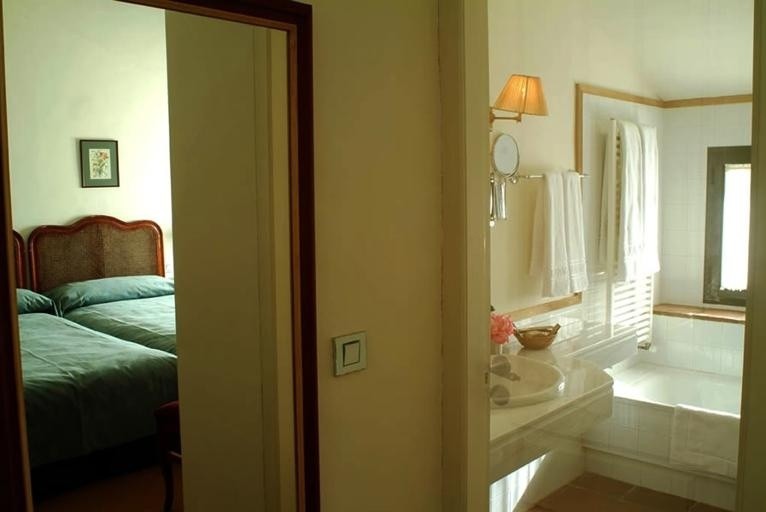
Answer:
[488,353,565,406]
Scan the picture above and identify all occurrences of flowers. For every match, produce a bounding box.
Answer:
[489,308,514,345]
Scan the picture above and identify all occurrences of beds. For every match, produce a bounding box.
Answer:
[28,213,177,354]
[11,229,178,470]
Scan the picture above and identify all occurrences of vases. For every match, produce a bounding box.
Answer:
[494,342,503,356]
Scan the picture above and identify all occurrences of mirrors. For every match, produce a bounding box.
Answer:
[492,133,521,179]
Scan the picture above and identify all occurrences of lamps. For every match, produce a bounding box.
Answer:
[489,74,550,126]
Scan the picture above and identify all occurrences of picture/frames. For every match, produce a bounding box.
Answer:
[80,139,120,187]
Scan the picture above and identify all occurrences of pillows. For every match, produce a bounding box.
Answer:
[16,287,52,314]
[47,275,175,311]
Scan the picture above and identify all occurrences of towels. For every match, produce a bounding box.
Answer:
[528,171,571,297]
[669,403,740,481]
[562,171,590,294]
[596,119,644,283]
[637,123,661,277]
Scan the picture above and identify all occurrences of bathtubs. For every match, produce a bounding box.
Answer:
[582,362,742,509]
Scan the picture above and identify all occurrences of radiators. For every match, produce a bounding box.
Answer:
[606,118,654,342]
